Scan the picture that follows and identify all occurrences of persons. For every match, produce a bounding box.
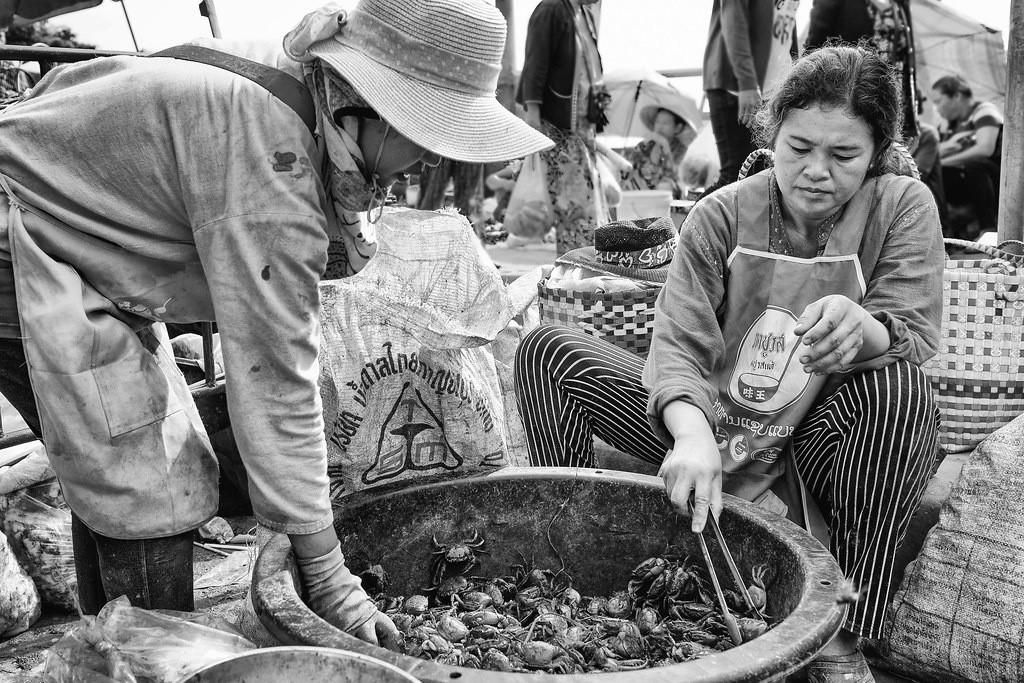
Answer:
[515,0,610,258]
[802,0,920,138]
[415,154,486,249]
[697,0,800,202]
[514,47,945,683]
[0,0,557,651]
[485,159,523,237]
[912,74,1004,239]
[622,102,699,201]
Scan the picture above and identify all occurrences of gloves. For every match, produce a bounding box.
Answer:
[300,539,400,653]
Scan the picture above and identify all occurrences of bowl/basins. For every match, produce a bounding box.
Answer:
[176,646,424,683]
[250,466,851,683]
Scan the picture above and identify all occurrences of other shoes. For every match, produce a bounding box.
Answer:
[806,645,876,683]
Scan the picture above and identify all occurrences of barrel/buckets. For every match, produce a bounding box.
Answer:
[616,190,673,222]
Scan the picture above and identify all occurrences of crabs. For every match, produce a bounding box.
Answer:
[356,528,777,674]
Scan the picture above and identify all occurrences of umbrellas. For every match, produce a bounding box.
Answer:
[605,68,704,144]
[910,0,1006,109]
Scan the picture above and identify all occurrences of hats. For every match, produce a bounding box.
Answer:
[639,103,698,145]
[556,215,679,287]
[307,0,557,163]
